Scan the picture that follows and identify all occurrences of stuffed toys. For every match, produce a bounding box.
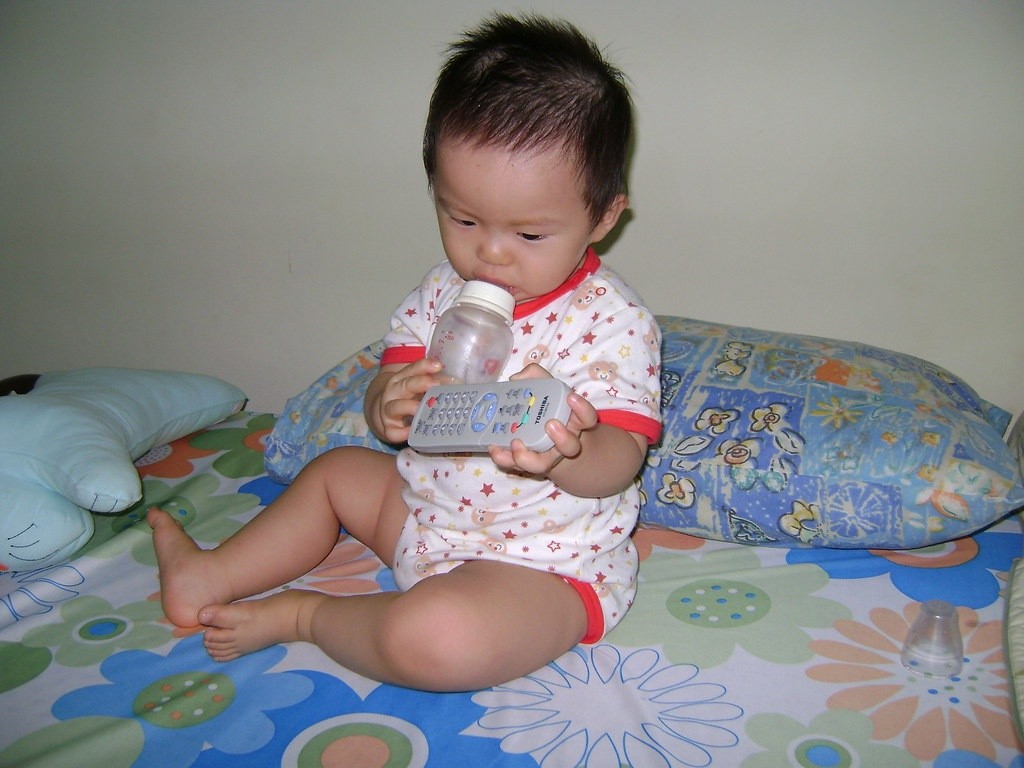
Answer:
[0,368,246,574]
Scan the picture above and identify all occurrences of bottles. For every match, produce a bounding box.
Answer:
[402,279,517,426]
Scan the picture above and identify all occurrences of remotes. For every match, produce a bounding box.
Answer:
[407,376,573,454]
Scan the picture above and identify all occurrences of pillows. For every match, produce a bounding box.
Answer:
[262,317,1023,550]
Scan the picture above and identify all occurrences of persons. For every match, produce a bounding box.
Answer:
[144,14,663,693]
